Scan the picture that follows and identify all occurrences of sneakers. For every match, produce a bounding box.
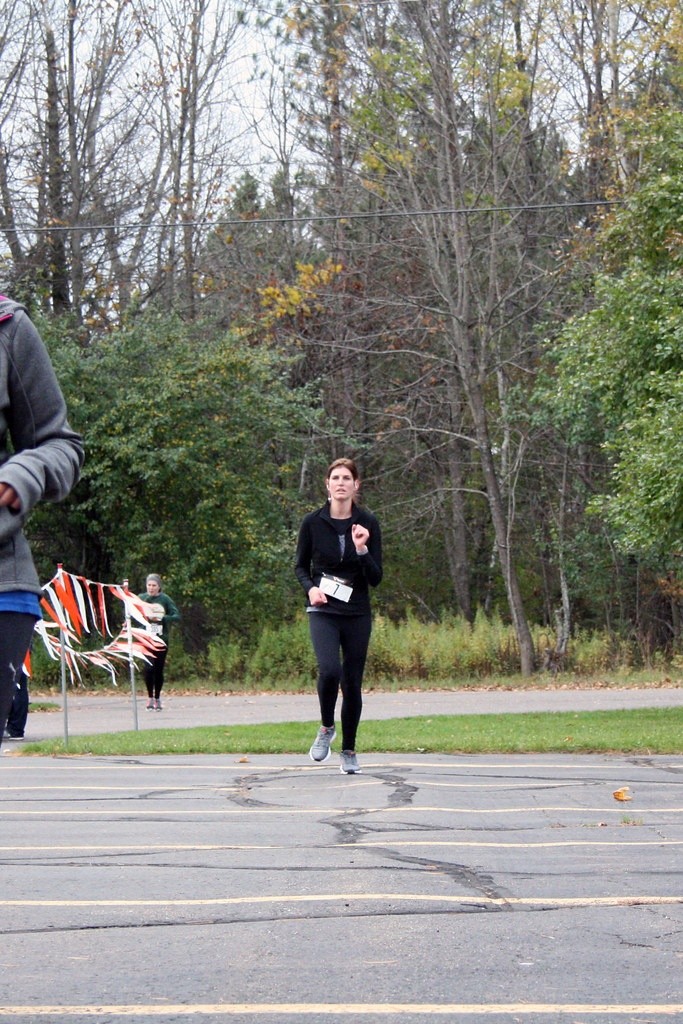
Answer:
[339,750,362,774]
[154,698,162,712]
[146,698,154,712]
[309,723,337,763]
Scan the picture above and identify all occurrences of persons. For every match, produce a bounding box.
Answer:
[3,639,32,741]
[294,458,383,774]
[0,295,85,743]
[131,574,179,710]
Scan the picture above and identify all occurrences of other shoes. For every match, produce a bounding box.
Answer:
[1,728,24,741]
